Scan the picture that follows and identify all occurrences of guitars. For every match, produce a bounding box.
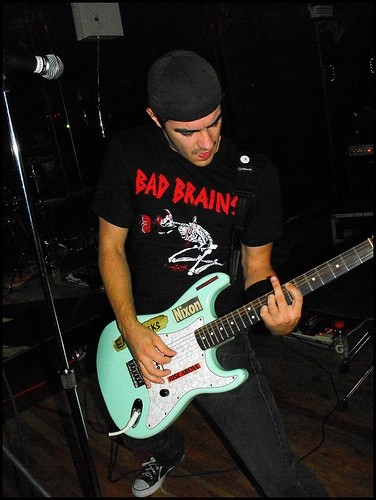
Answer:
[96,236,376,441]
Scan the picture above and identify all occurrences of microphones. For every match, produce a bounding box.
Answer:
[0,54,64,80]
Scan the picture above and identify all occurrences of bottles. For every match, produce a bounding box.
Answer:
[329,321,347,367]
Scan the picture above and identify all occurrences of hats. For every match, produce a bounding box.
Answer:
[146,49,222,121]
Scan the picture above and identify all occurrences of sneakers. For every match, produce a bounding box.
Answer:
[131,449,185,498]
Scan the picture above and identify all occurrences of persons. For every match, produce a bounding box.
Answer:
[98,49,329,500]
